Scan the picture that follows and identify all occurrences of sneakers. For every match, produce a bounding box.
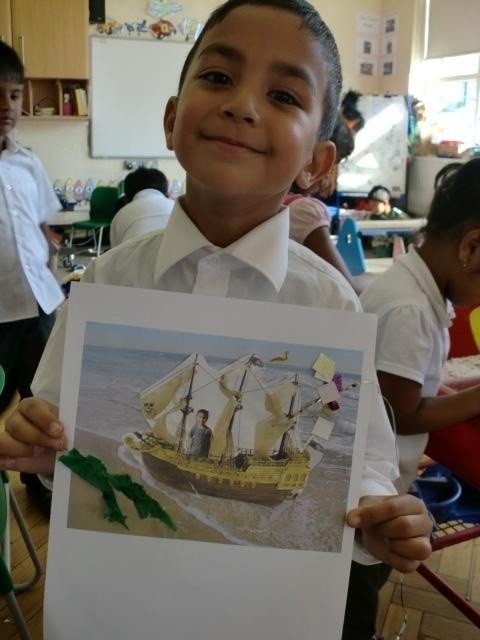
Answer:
[25,476,52,517]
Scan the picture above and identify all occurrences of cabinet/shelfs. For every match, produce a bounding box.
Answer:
[0,0,90,120]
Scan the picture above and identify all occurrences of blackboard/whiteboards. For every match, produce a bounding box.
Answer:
[90,35,195,158]
[337,94,408,199]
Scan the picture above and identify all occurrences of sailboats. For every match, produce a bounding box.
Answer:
[119,347,360,508]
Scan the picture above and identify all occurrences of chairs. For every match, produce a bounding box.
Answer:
[1,354,42,640]
[64,181,125,259]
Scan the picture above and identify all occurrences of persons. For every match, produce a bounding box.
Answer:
[0,0,439,640]
[341,156,480,639]
[187,409,214,458]
[109,165,176,251]
[366,186,421,257]
[303,162,338,199]
[0,41,67,417]
[282,112,380,296]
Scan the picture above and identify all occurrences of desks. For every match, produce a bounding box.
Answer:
[44,210,89,258]
[341,219,434,254]
